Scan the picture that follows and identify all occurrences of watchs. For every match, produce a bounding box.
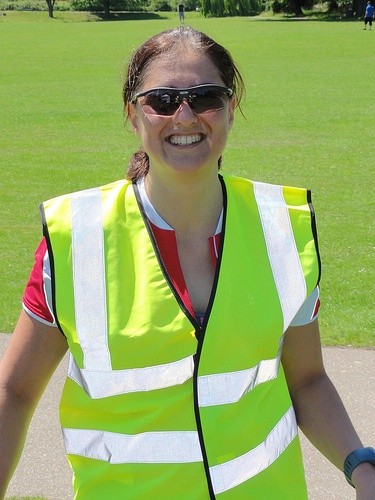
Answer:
[344,447,375,488]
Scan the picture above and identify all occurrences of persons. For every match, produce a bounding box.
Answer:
[0,26,375,500]
[177,1,186,24]
[363,0,375,30]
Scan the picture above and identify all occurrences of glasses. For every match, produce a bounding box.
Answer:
[131,84,233,116]
